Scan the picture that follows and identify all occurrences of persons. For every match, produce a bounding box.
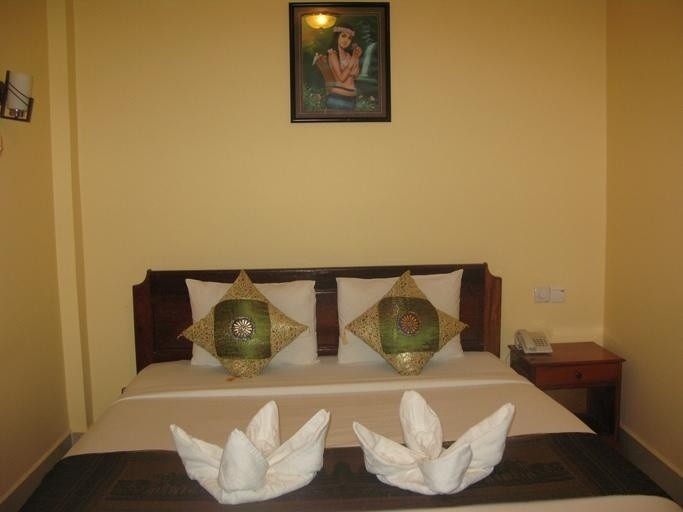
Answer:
[323,23,361,114]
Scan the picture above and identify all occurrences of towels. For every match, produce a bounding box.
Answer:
[352,389,516,495]
[168,399,331,505]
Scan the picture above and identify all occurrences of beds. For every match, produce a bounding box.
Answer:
[17,262,683,512]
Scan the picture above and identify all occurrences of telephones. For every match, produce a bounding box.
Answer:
[516,329,552,354]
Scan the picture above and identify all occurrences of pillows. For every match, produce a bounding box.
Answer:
[335,269,465,367]
[343,270,470,376]
[184,278,320,367]
[175,268,310,382]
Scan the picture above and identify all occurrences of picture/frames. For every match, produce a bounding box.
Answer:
[288,2,391,123]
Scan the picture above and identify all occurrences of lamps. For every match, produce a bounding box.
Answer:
[1,70,34,123]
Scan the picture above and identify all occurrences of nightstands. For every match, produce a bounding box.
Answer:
[507,341,626,433]
